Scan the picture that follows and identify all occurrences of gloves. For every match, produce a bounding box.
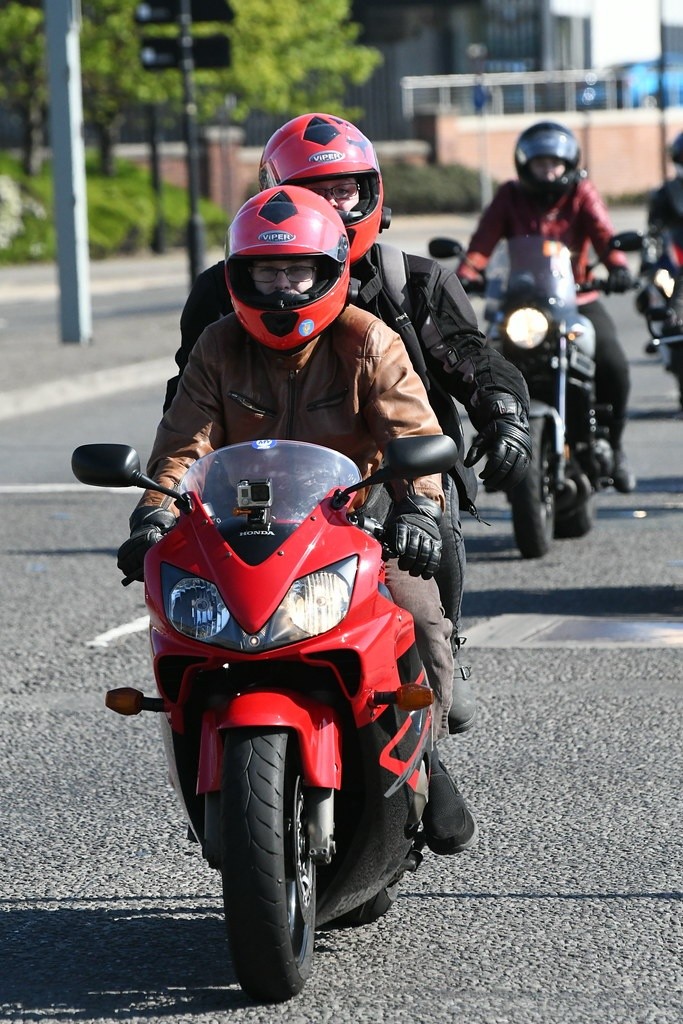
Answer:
[461,392,532,494]
[454,252,488,296]
[384,496,442,580]
[607,252,631,294]
[115,505,174,583]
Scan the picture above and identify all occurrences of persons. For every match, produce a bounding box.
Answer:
[156,108,537,735]
[92,184,482,857]
[642,129,683,355]
[419,121,642,499]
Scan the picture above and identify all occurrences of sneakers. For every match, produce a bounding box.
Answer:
[422,760,479,854]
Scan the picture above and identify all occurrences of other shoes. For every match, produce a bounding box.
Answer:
[611,449,635,492]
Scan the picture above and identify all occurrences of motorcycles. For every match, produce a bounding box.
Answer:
[638,224,683,405]
[428,230,646,559]
[71,434,459,1002]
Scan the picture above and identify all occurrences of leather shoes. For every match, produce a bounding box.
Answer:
[447,658,479,733]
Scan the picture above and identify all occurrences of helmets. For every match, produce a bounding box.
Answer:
[515,123,580,193]
[257,112,386,269]
[224,184,351,352]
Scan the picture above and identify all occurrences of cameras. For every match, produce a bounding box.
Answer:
[233,478,274,527]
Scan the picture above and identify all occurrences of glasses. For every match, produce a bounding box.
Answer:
[309,182,360,198]
[248,265,319,284]
